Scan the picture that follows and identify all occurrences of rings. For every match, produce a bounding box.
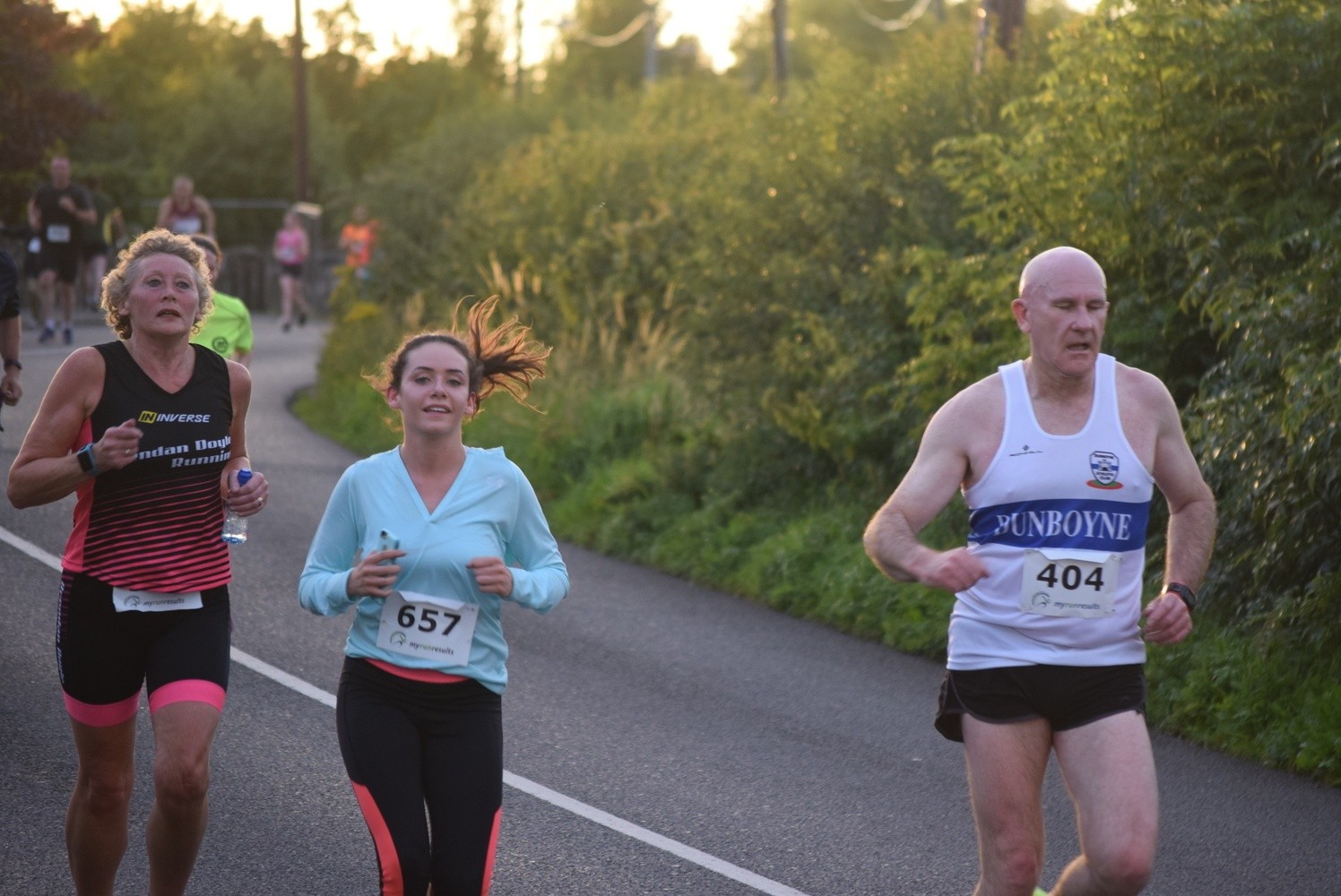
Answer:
[125,448,130,456]
[258,497,263,507]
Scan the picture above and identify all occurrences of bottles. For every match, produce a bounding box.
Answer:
[221,466,252,545]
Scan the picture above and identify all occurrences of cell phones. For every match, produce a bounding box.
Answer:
[376,530,399,589]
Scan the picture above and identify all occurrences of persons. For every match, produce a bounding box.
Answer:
[24,155,378,368]
[862,245,1217,896]
[0,251,23,406]
[296,295,571,896]
[5,229,268,896]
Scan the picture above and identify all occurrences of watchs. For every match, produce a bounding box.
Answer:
[77,443,101,477]
[1161,582,1197,613]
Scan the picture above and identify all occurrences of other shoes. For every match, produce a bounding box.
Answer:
[62,329,72,344]
[298,313,308,326]
[283,322,293,332]
[39,327,55,342]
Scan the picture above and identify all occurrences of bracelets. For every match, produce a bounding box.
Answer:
[4,359,22,371]
